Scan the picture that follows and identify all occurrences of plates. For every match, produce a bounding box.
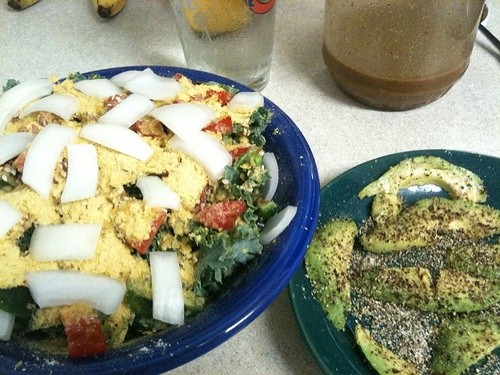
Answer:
[287,148,500,375]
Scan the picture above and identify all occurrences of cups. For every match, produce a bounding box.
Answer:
[322,0,488,112]
[169,0,276,92]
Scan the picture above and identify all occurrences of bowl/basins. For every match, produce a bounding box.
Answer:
[0,65,319,375]
